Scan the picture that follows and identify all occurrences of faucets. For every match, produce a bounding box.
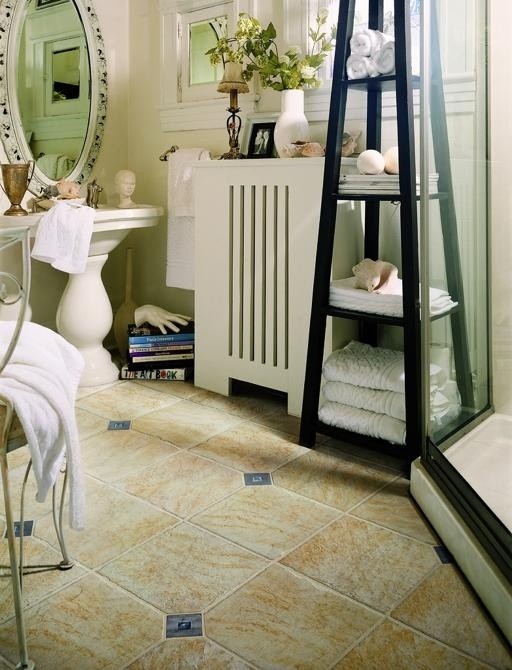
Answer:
[87,179,103,208]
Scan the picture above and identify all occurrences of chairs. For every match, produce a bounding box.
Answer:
[0,226,71,670]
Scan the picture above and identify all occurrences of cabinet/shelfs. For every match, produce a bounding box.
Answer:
[297,0,475,480]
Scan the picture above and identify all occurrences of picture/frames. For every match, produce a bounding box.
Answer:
[247,122,276,158]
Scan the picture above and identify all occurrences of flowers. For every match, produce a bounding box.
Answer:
[205,12,337,91]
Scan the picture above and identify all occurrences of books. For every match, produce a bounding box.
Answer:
[120,320,195,381]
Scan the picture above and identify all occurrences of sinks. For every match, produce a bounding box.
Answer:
[0,204,164,256]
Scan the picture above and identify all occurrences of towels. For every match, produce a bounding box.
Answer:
[328,258,459,321]
[35,153,74,182]
[30,196,96,275]
[0,319,87,532]
[345,28,396,80]
[165,149,214,291]
[318,340,452,445]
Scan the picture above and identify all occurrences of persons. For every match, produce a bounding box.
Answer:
[134,304,192,335]
[253,128,270,154]
[114,169,138,208]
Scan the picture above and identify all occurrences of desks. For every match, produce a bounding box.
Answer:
[190,154,364,418]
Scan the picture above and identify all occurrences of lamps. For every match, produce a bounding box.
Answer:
[216,60,250,159]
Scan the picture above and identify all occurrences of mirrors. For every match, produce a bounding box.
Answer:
[0,0,109,199]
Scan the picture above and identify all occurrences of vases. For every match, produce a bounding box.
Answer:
[273,89,311,158]
